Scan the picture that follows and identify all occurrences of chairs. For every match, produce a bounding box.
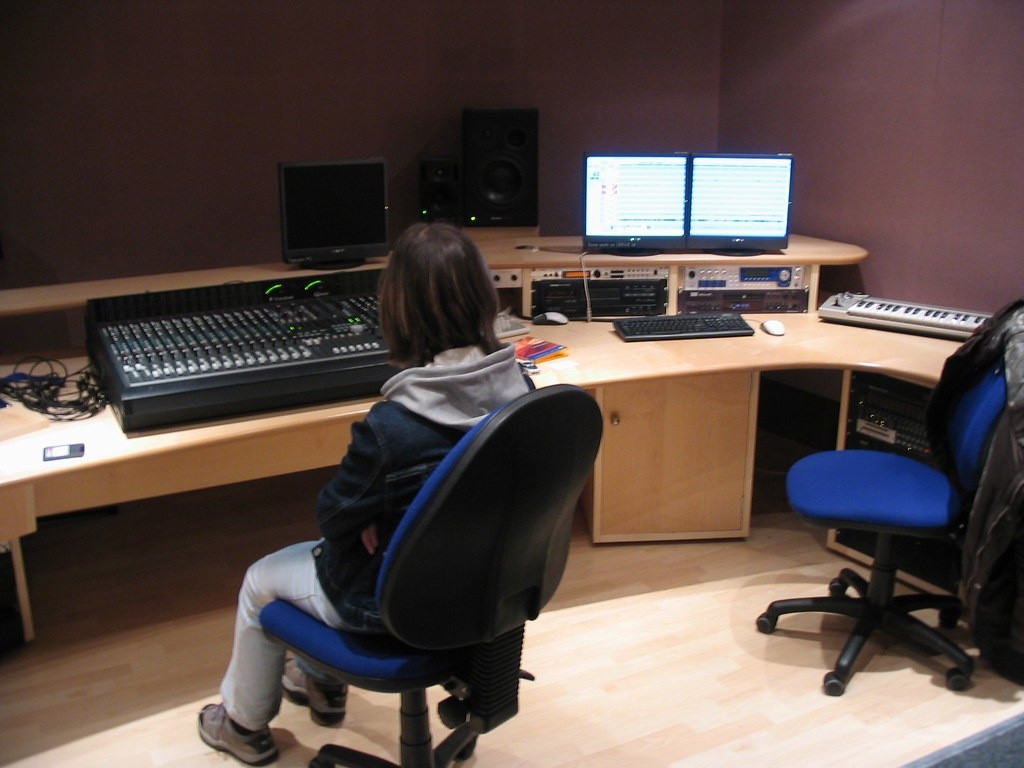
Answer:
[755,348,1007,696]
[259,382,604,768]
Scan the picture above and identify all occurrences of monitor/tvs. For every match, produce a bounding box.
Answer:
[686,153,794,257]
[277,159,390,270]
[582,152,690,256]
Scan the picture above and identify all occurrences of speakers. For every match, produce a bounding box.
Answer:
[417,156,460,227]
[461,106,539,228]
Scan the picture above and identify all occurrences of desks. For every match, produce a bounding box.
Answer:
[0,234,973,644]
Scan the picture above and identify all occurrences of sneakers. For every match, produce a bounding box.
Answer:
[281,658,348,725]
[198,703,278,763]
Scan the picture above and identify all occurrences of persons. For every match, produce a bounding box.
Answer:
[197,222,536,767]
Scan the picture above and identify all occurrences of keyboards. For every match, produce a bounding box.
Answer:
[494,316,531,339]
[614,314,754,342]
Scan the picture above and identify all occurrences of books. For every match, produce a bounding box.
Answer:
[515,336,569,364]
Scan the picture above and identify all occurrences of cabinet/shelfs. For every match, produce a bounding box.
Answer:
[577,370,759,543]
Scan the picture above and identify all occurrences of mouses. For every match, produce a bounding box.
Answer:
[761,320,785,336]
[533,312,569,325]
